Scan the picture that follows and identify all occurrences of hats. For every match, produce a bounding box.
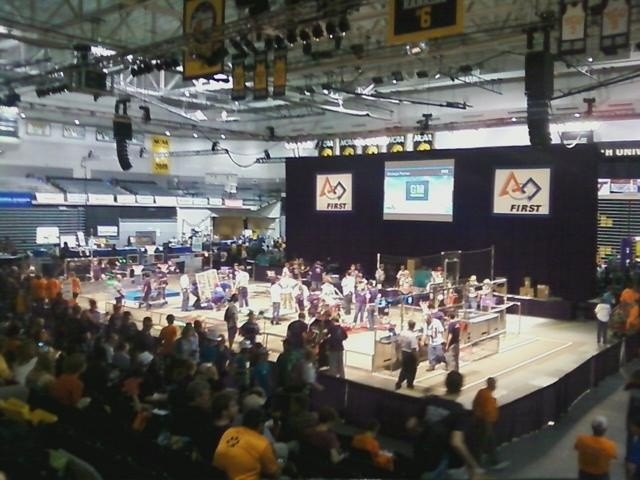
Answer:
[592,415,608,430]
[242,393,268,412]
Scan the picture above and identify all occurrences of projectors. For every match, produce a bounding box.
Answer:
[64,63,107,96]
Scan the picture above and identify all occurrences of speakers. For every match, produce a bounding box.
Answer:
[524,49,552,145]
[112,114,132,171]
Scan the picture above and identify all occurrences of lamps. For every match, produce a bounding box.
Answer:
[229,16,351,53]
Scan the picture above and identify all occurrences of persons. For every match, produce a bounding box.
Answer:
[1,238,640,479]
